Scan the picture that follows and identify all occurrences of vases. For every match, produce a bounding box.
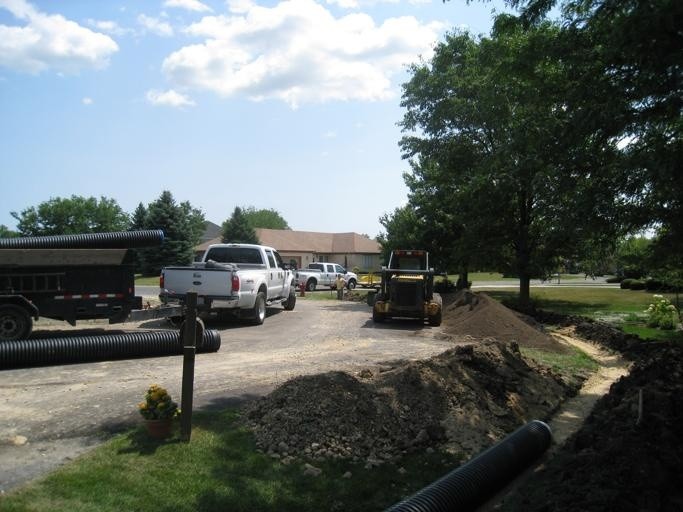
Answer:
[140,419,171,440]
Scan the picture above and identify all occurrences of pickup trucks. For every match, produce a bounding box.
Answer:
[158,242,297,325]
[293,261,357,291]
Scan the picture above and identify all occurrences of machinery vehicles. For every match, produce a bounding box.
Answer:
[371,249,445,326]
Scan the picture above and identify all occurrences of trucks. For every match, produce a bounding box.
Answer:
[0,247,144,344]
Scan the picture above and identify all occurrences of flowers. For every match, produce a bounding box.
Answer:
[137,384,181,419]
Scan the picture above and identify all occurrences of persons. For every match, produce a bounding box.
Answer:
[332,274,347,300]
